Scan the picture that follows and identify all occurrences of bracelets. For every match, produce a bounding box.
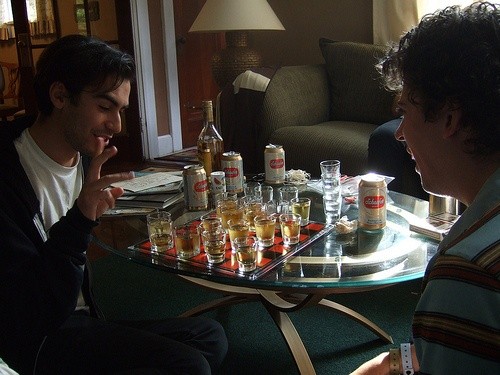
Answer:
[400,343,414,375]
[389,347,401,375]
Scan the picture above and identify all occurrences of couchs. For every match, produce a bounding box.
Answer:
[260,35,430,200]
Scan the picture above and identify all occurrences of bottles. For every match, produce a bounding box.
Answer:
[197,101,224,194]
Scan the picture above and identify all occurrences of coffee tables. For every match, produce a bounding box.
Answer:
[96,164,464,375]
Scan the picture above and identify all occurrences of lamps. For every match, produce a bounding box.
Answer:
[187,0,287,97]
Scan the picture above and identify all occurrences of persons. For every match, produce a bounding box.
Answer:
[0,35,229,375]
[348,0,500,375]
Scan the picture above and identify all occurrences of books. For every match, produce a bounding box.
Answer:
[151,149,199,167]
[99,171,184,217]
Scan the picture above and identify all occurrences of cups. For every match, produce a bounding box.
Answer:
[228,219,249,250]
[215,193,237,214]
[278,187,298,213]
[146,211,173,252]
[254,215,277,247]
[266,200,288,230]
[234,236,256,272]
[290,197,310,225]
[173,222,200,259]
[320,160,342,226]
[202,230,226,264]
[240,195,257,213]
[253,186,273,206]
[278,213,302,245]
[243,182,260,200]
[200,214,221,231]
[221,205,243,233]
[244,202,262,233]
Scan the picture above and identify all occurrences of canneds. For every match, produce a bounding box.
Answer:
[221,151,243,194]
[265,143,285,184]
[182,165,208,211]
[357,175,387,230]
